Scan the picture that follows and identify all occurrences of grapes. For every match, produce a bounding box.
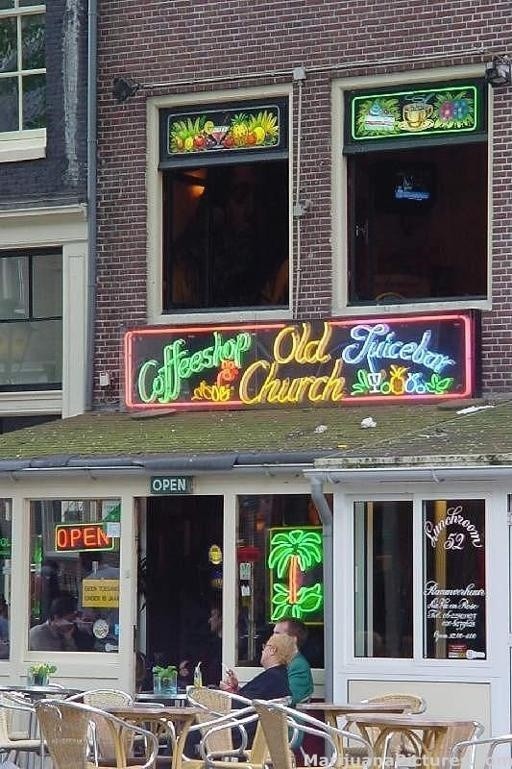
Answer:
[439,99,471,121]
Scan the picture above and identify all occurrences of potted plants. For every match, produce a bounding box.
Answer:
[26,663,57,686]
[152,665,178,697]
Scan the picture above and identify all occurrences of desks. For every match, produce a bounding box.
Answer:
[345,712,476,769]
[0,685,67,703]
[101,706,197,769]
[133,694,188,709]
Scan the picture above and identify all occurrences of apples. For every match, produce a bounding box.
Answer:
[223,137,234,147]
[194,135,206,145]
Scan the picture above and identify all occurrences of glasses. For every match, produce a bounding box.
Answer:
[262,644,270,649]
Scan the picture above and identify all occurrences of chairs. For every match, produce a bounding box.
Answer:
[182,694,293,769]
[187,686,298,769]
[0,691,50,769]
[252,699,374,769]
[342,692,426,761]
[63,688,134,708]
[33,699,159,769]
[431,720,485,769]
[449,734,512,769]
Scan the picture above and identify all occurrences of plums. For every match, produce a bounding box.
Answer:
[205,136,217,149]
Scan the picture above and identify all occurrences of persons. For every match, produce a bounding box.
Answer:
[29,595,76,651]
[229,618,314,750]
[159,634,296,765]
[178,606,246,689]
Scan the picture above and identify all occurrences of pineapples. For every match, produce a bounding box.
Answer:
[250,110,279,145]
[170,115,207,150]
[231,113,251,146]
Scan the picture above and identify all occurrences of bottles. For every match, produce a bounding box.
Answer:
[194,667,202,688]
